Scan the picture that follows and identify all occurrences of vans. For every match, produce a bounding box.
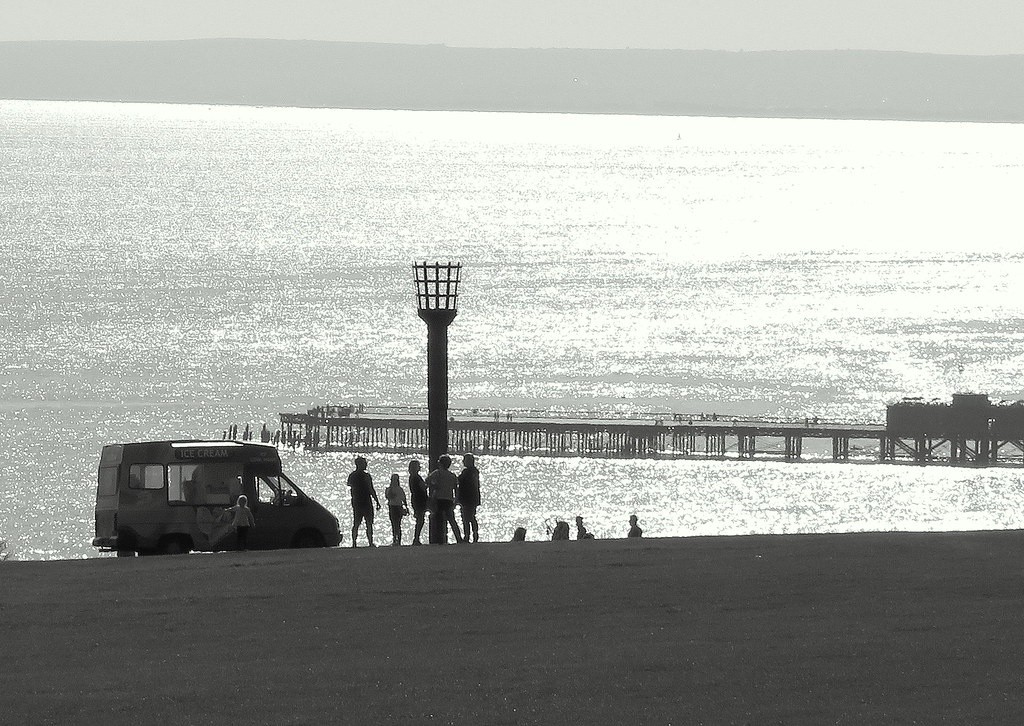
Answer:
[94,437,345,557]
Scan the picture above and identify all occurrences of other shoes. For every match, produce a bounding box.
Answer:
[413,540,422,546]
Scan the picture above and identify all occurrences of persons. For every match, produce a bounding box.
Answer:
[628,515,642,537]
[552,521,569,541]
[408,453,481,545]
[214,494,256,553]
[575,516,586,540]
[347,457,381,547]
[512,526,528,541]
[386,473,410,546]
